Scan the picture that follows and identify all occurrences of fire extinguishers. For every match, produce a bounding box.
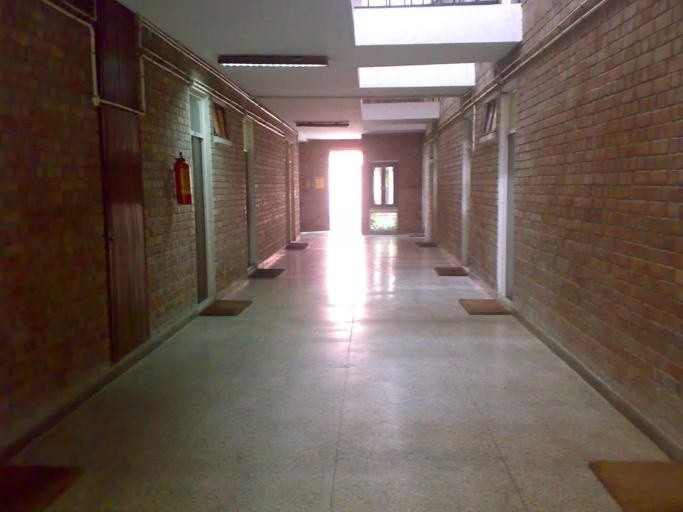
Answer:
[174,151,192,204]
